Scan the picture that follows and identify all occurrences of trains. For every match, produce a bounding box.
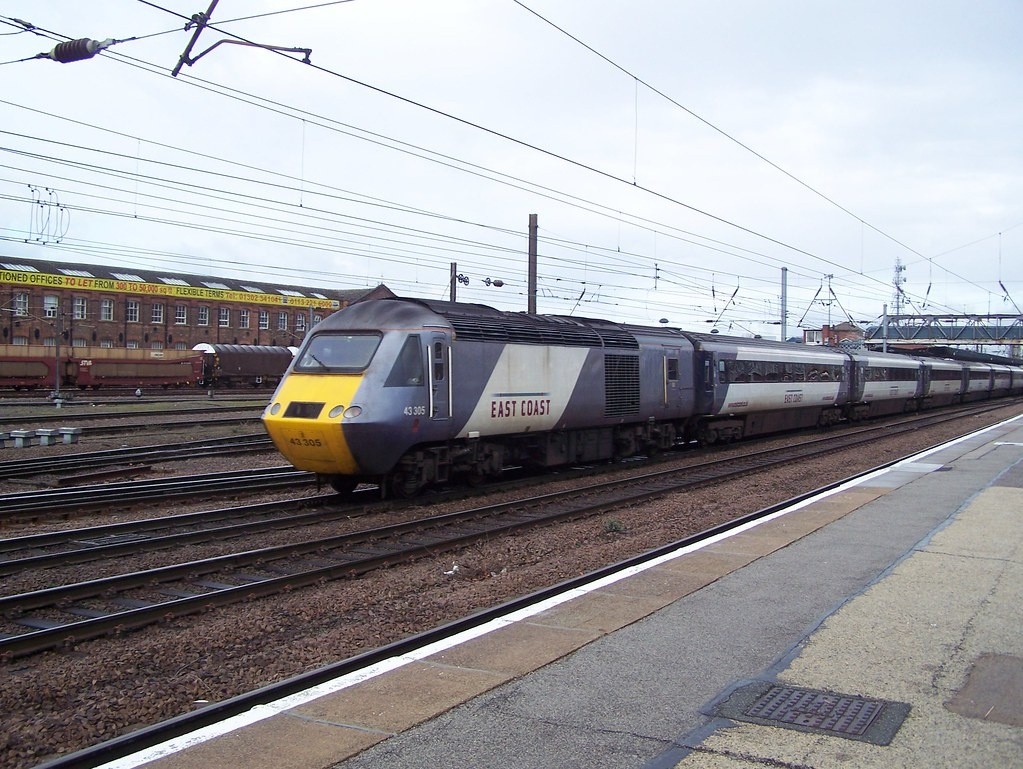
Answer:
[262,297,1023,500]
[0,342,300,394]
